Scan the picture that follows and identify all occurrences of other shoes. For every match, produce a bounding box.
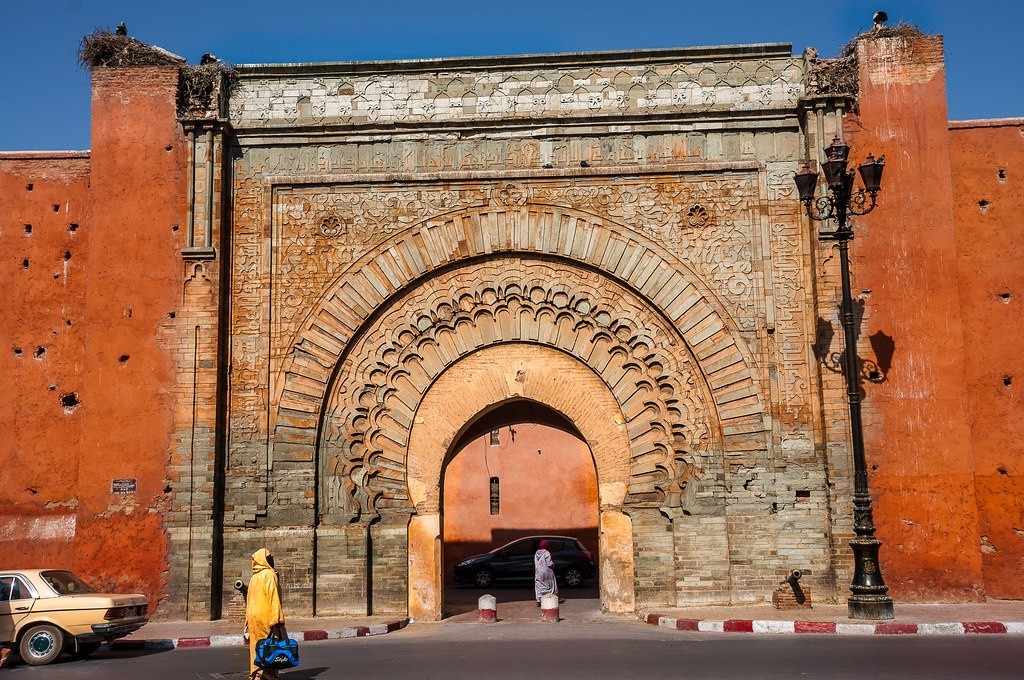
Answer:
[559,598,565,604]
[537,602,541,606]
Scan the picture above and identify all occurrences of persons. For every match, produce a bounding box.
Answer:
[534,540,565,606]
[246,547,286,680]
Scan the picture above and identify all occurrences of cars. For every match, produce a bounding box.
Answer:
[456,535,595,595]
[0,568,151,664]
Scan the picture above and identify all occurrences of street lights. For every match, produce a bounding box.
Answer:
[794,136,899,618]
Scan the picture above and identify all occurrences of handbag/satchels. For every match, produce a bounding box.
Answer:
[255,623,299,670]
